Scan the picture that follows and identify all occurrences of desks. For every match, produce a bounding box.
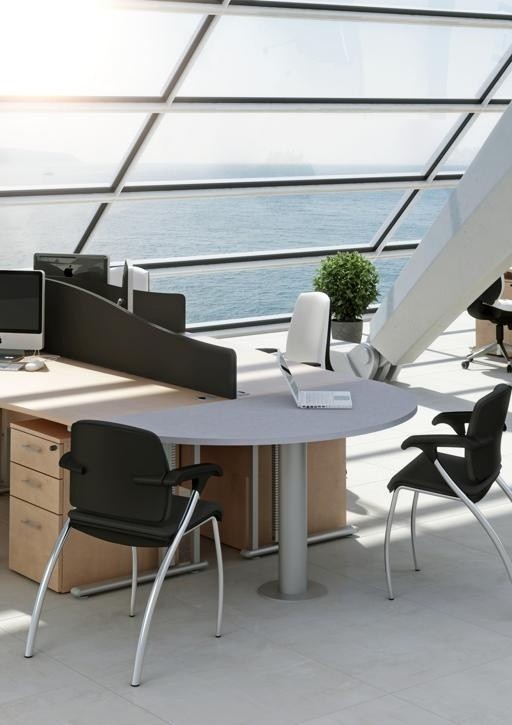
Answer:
[0,328,417,605]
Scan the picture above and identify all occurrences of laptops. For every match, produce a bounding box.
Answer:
[277,349,353,409]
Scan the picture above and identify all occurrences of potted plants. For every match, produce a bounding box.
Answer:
[313,249,382,344]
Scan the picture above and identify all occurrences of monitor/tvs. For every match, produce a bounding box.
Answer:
[133,290,187,334]
[116,258,134,314]
[34,253,110,293]
[0,269,45,361]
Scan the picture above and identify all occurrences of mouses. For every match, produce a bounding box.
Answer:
[25,362,45,371]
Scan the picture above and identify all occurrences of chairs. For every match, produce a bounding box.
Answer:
[283,293,335,370]
[383,382,510,603]
[24,417,225,688]
[462,277,512,374]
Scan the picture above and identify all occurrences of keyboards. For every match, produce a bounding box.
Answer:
[0,363,25,371]
